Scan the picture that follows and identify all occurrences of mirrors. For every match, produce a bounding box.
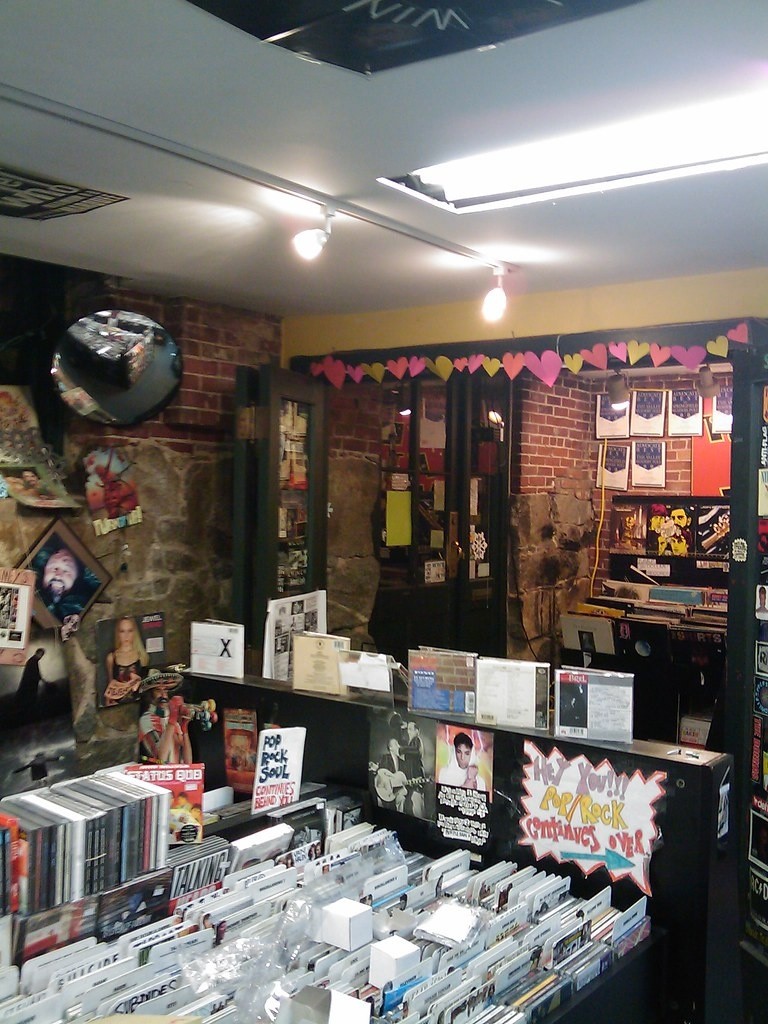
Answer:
[42,304,185,428]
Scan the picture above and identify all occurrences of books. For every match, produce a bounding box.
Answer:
[560,580,728,665]
[4,772,232,971]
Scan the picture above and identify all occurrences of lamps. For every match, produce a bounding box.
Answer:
[482,267,509,321]
[696,363,720,398]
[294,205,335,260]
[605,369,629,410]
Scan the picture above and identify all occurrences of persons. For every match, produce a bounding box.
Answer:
[439,732,488,794]
[374,713,426,819]
[38,548,81,619]
[105,618,153,705]
[18,649,44,711]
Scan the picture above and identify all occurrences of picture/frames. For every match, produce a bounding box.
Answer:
[13,515,114,643]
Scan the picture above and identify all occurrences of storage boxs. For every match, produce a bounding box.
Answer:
[323,898,372,951]
[368,934,421,988]
[292,985,371,1024]
